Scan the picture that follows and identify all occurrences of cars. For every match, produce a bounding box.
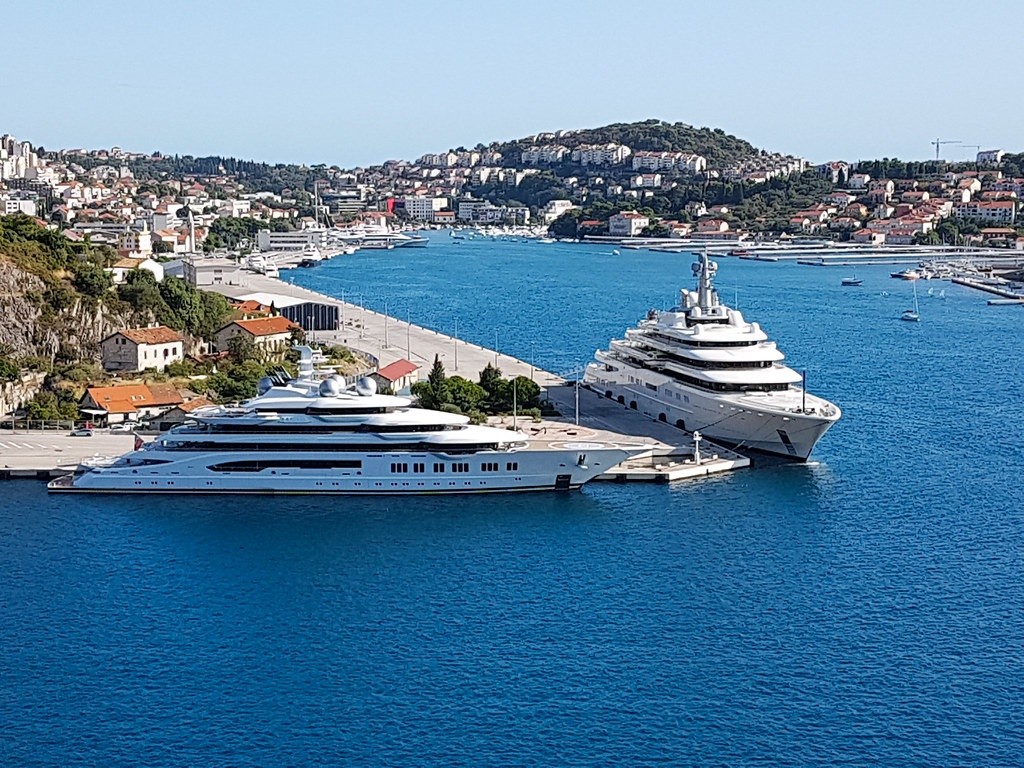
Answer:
[77,422,93,429]
[70,429,92,437]
[123,422,137,429]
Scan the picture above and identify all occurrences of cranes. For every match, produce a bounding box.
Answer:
[929,138,962,161]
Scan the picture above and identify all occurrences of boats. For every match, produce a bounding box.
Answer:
[581,232,843,468]
[241,199,580,281]
[901,279,919,320]
[46,340,661,497]
[841,265,867,286]
[889,230,1024,291]
